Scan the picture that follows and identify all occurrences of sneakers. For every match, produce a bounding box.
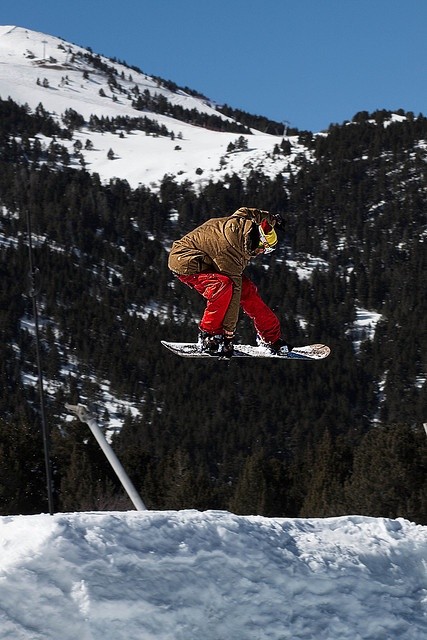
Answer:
[197,333,224,356]
[256,334,293,356]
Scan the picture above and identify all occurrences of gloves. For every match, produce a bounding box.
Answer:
[217,328,236,361]
[269,214,282,225]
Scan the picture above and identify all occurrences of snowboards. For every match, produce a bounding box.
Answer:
[160,340,331,360]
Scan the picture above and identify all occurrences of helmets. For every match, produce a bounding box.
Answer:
[255,219,278,255]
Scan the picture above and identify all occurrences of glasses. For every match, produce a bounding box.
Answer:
[258,225,277,255]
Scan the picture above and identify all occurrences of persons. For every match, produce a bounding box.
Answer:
[167,207,293,357]
